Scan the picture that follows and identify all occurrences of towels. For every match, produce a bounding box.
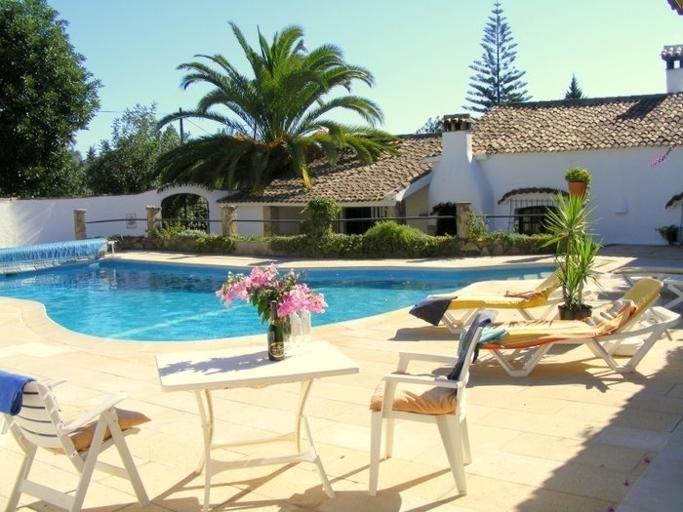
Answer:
[1,371,32,416]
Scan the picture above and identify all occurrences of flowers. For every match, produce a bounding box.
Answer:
[216,261,331,320]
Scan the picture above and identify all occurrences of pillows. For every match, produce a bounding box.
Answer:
[370,369,457,417]
[59,400,151,450]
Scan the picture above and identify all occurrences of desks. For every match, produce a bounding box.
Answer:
[154,338,361,511]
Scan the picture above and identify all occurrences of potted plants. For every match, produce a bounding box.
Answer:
[540,189,605,321]
[564,168,592,197]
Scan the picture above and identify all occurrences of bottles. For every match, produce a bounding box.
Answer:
[267,300,285,362]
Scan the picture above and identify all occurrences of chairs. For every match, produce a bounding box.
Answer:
[408,260,602,339]
[0,369,150,512]
[473,277,672,375]
[366,312,493,502]
